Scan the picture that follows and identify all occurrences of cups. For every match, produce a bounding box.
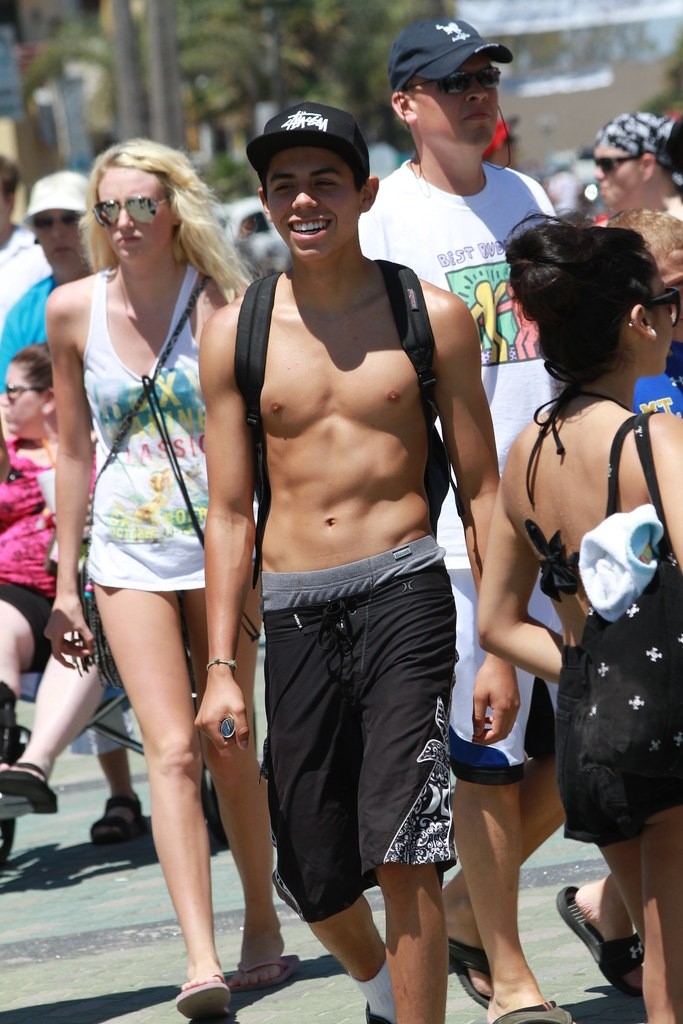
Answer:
[37,472,56,514]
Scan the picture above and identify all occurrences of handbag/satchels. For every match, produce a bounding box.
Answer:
[578,410,683,782]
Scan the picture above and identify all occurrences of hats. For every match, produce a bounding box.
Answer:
[387,18,514,92]
[22,170,91,233]
[246,102,372,180]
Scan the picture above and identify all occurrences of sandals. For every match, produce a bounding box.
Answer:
[90,792,146,846]
[0,762,57,814]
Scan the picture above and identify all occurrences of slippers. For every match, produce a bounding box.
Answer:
[492,1002,572,1024]
[229,955,300,993]
[557,887,644,997]
[175,969,230,1019]
[446,935,492,1009]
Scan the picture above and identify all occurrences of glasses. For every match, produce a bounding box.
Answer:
[28,211,81,230]
[93,197,170,229]
[405,66,501,95]
[5,383,51,403]
[592,156,641,175]
[611,286,681,331]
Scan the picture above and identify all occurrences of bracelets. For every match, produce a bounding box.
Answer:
[206,658,237,672]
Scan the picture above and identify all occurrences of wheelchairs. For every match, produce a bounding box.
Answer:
[0,663,227,870]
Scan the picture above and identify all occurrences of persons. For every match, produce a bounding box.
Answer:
[478,225,683,1024]
[45,139,300,1020]
[139,198,157,215]
[357,16,576,1024]
[0,157,147,845]
[542,112,683,221]
[554,207,683,989]
[98,204,112,225]
[193,101,520,1024]
